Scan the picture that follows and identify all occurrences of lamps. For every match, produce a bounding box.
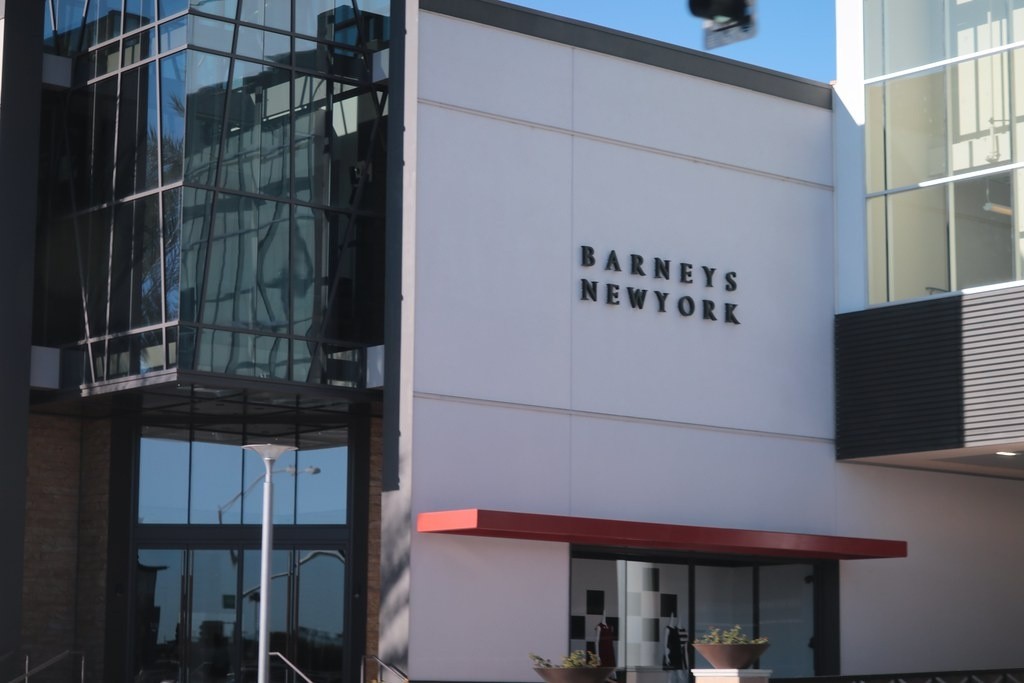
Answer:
[982,179,1012,216]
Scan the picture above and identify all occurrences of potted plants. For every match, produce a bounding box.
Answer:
[529,650,616,683]
[691,625,770,669]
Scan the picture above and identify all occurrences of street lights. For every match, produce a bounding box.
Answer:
[238,440,301,683]
[217,465,322,525]
